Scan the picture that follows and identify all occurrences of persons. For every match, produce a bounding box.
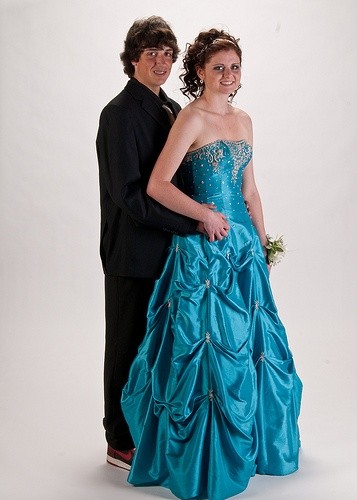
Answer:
[119,28,303,500]
[97,15,252,473]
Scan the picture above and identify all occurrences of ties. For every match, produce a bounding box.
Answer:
[162,104,175,126]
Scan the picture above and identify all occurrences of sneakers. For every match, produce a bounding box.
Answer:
[106,443,136,470]
